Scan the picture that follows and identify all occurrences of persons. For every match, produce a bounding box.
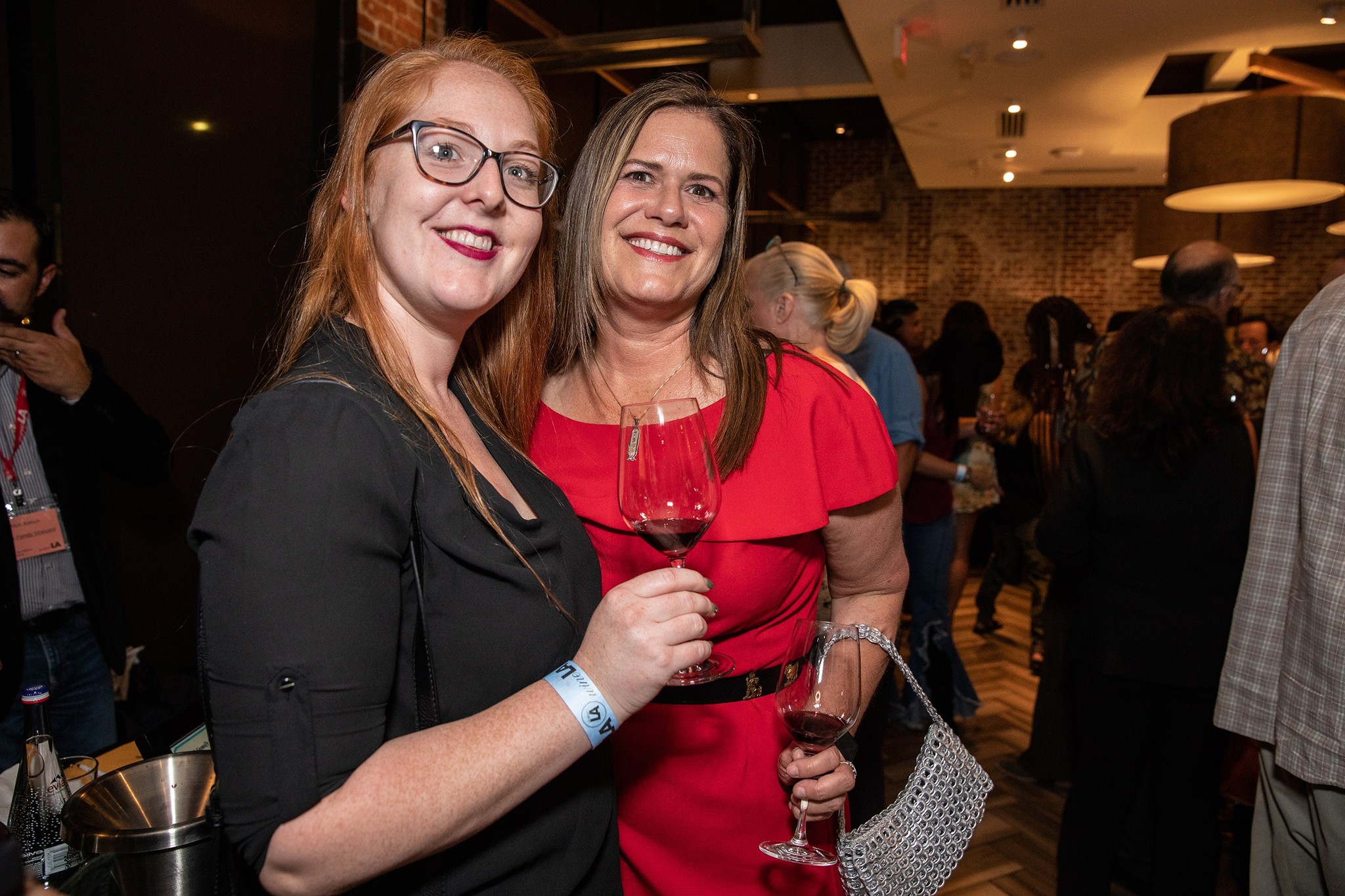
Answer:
[185,33,718,896]
[507,74,908,896]
[1231,314,1276,376]
[838,329,928,499]
[737,238,882,405]
[999,513,1075,779]
[1211,265,1345,896]
[903,349,993,729]
[1155,238,1242,319]
[911,301,1004,636]
[0,199,153,766]
[998,295,1110,676]
[1038,307,1260,896]
[881,297,921,344]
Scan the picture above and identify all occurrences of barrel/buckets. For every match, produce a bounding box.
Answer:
[59,749,217,895]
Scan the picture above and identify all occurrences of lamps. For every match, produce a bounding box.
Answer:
[1324,198,1344,236]
[1163,94,1345,214]
[1131,212,1281,271]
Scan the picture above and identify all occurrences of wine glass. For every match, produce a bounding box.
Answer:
[617,399,738,687]
[756,618,865,867]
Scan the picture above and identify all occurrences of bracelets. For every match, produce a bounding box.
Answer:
[953,462,970,483]
[543,661,619,751]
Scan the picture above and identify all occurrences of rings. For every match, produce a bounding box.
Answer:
[838,760,858,779]
[14,350,21,359]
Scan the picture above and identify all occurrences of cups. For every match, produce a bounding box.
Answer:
[58,756,98,796]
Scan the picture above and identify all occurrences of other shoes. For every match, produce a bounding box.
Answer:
[973,618,1002,635]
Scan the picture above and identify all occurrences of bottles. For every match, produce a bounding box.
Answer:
[4,688,72,889]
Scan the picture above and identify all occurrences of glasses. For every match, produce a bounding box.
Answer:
[364,119,565,210]
[765,235,798,289]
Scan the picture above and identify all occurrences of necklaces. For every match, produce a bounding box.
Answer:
[589,344,697,464]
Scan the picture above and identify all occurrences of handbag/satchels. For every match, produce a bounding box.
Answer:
[816,621,995,896]
